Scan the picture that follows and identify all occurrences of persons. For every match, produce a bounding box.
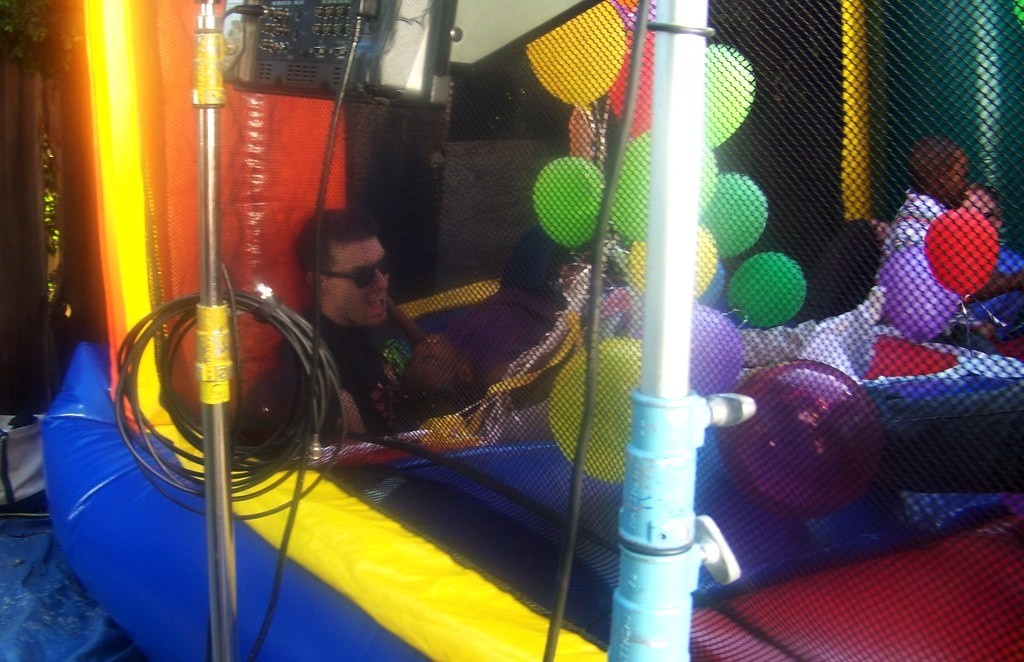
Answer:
[837,136,1024,360]
[277,207,888,445]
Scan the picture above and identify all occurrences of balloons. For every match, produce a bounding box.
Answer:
[875,245,964,347]
[926,209,998,301]
[525,0,887,515]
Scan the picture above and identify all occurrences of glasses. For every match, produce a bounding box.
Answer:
[321,256,392,288]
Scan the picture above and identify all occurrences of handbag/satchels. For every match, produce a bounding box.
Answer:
[0,414,44,507]
